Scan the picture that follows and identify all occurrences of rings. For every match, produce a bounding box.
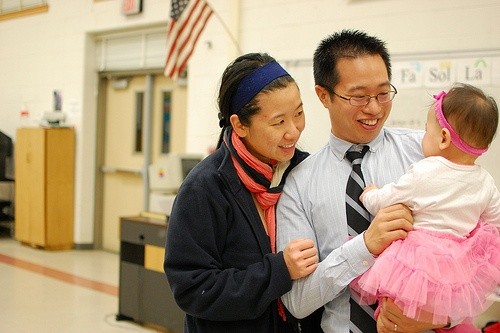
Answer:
[394,323,398,332]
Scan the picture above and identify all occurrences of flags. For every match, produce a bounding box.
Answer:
[165,0,216,79]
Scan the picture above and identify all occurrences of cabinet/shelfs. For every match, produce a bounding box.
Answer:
[115,216,186,333]
[14,127,75,251]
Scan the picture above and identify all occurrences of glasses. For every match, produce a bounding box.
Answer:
[323,84,397,106]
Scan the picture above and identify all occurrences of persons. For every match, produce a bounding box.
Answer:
[348,83,500,333]
[164,53,325,333]
[275,30,500,333]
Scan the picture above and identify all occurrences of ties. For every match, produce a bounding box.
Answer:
[343,145,379,333]
